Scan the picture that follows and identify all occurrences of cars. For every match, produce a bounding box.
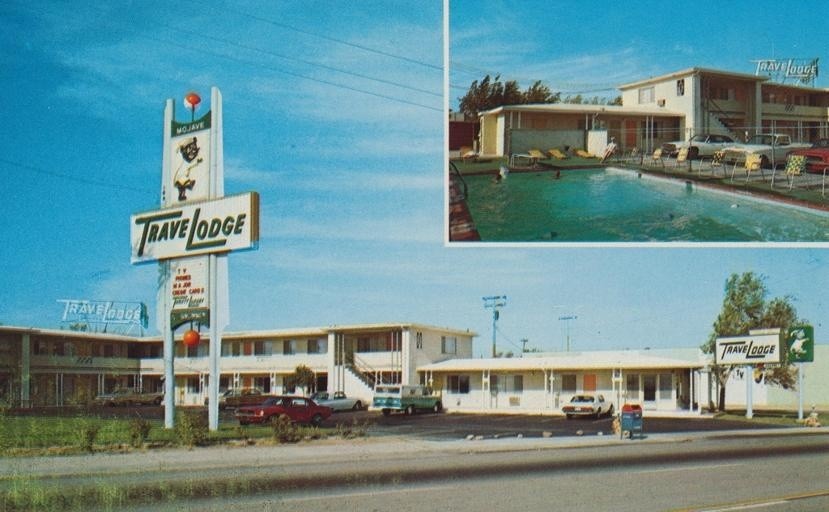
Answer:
[785,137,829,175]
[662,133,740,159]
[562,392,614,419]
[95,387,164,407]
[204,387,363,426]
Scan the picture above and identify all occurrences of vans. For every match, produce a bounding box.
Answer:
[372,385,440,415]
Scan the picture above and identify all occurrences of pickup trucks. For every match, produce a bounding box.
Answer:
[720,133,811,170]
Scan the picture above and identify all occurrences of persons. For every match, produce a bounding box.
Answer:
[553,169,562,179]
[490,171,504,181]
[597,135,621,165]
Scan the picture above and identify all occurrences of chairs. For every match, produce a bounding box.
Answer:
[458,146,478,163]
[650,145,809,191]
[527,147,599,161]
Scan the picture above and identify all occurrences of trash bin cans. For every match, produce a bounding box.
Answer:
[621,404,642,439]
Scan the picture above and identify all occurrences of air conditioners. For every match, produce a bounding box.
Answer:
[658,99,665,106]
